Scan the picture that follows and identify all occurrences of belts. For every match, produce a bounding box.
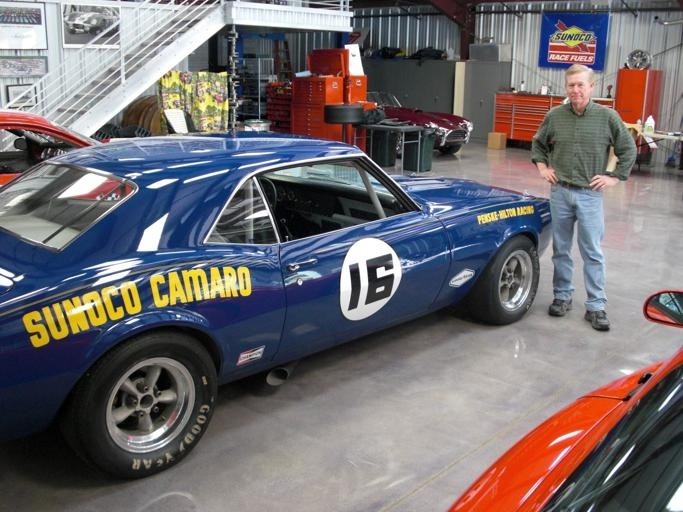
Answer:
[559,182,583,190]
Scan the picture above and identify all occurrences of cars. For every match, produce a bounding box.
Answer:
[65,7,117,35]
[365,87,472,155]
[448,285,683,511]
[1,112,122,174]
[0,141,560,476]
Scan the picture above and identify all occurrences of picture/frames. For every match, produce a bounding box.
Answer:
[1,1,122,111]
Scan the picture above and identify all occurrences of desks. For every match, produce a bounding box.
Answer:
[352,119,423,177]
[636,128,683,173]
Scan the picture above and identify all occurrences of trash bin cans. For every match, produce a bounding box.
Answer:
[403,125,436,171]
[366,122,400,166]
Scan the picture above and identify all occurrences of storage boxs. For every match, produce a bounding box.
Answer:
[487,132,507,149]
[289,46,377,152]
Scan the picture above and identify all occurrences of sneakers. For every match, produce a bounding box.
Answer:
[585,311,610,331]
[549,298,572,316]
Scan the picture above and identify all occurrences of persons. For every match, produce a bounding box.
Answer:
[4,7,42,18]
[3,59,43,74]
[102,7,112,16]
[529,62,639,329]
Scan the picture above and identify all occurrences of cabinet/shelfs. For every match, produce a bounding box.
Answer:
[491,90,617,144]
[233,56,273,120]
